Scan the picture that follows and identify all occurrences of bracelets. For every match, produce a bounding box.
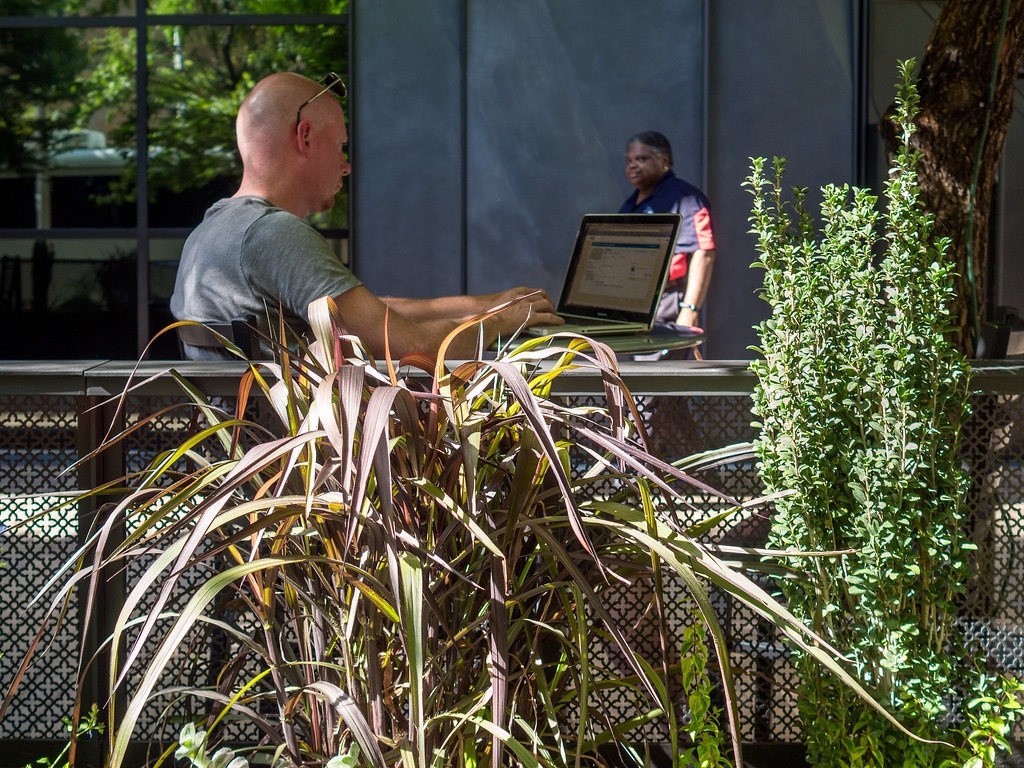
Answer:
[679,302,703,313]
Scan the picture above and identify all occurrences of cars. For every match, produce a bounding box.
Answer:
[225,149,238,162]
[25,127,107,153]
[17,149,128,174]
[119,146,187,167]
[201,144,229,158]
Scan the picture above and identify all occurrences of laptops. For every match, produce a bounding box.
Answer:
[521,212,683,335]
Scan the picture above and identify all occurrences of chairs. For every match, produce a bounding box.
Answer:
[685,304,1023,742]
[178,316,295,740]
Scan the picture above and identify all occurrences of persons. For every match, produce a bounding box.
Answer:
[169,71,565,360]
[624,130,725,495]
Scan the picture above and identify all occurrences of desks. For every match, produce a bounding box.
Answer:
[486,324,706,493]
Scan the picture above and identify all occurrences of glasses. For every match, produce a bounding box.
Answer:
[297,72,347,136]
